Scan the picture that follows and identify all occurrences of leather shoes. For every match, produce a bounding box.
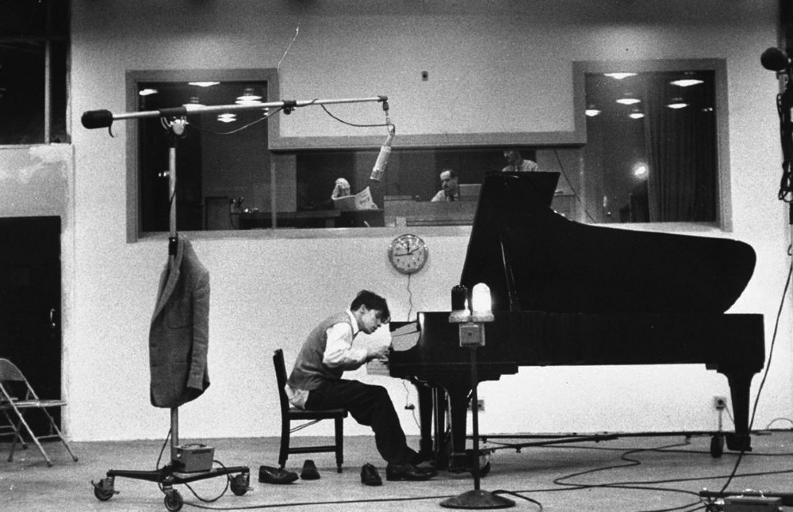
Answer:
[361,464,382,486]
[301,460,321,480]
[259,466,298,483]
[386,463,433,481]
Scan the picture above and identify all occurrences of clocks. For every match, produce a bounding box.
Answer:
[386,230,428,276]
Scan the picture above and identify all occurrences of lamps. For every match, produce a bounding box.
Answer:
[438,282,516,510]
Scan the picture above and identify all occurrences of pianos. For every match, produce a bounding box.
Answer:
[388,172,765,471]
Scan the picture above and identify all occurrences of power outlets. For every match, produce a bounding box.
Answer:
[714,395,726,410]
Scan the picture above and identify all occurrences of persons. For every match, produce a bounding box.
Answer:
[428,168,462,203]
[334,176,378,211]
[498,148,540,174]
[284,289,440,482]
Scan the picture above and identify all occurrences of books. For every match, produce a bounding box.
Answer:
[331,185,373,211]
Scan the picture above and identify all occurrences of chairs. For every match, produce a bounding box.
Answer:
[271,346,350,474]
[0,357,81,466]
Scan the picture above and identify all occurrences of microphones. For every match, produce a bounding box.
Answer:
[369,130,395,183]
